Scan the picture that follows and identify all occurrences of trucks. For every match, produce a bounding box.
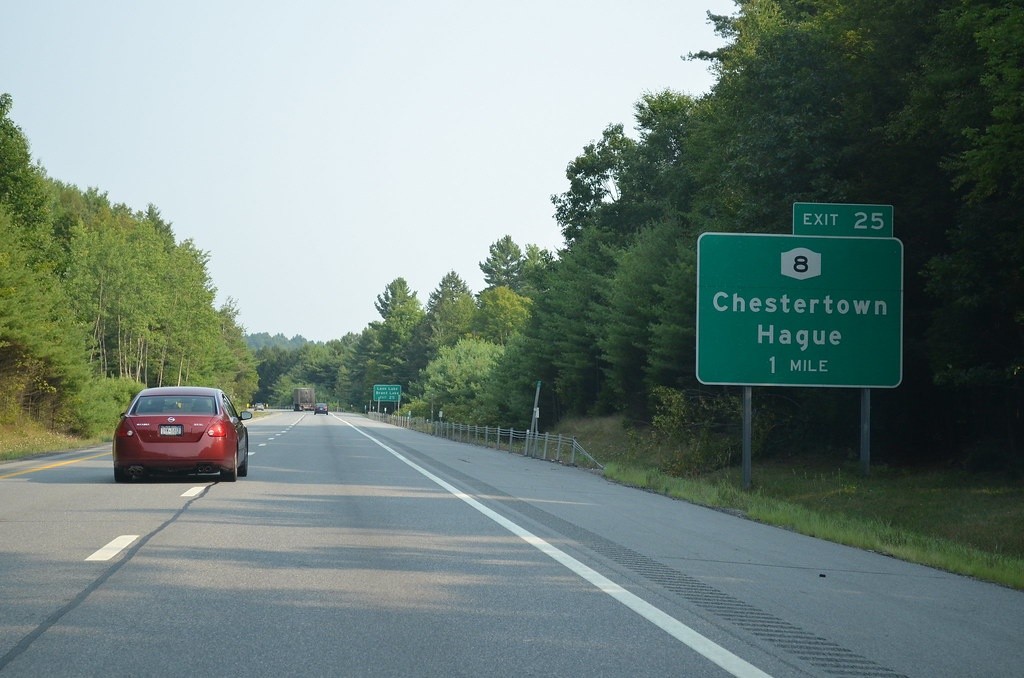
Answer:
[293,388,315,411]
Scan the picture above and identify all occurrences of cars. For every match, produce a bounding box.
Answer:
[254,403,264,411]
[113,387,252,482]
[314,403,328,415]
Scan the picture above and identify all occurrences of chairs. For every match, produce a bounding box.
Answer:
[191,399,211,414]
[149,399,166,413]
